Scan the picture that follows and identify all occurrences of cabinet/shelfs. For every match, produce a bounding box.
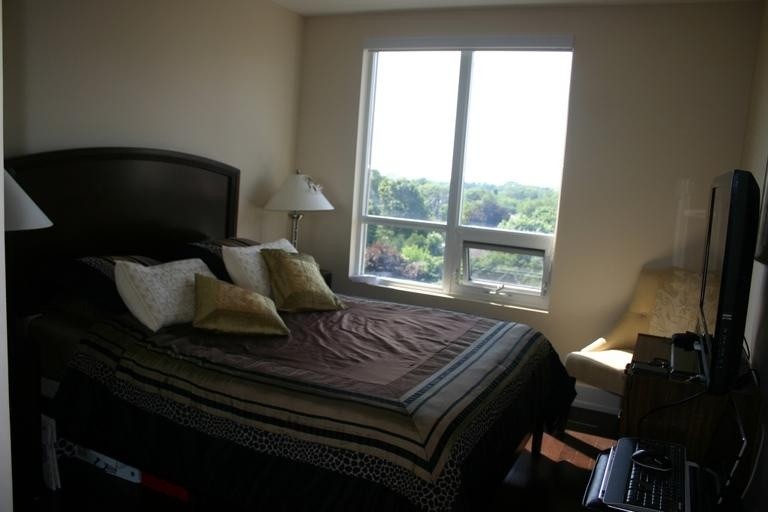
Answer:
[618,332,761,462]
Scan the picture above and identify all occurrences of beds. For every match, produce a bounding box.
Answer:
[4,147,550,512]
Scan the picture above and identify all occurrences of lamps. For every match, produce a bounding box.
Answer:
[4,170,56,233]
[263,169,335,259]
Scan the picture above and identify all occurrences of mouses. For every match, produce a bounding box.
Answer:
[632,445,676,475]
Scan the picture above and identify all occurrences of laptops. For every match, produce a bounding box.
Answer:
[602,394,747,512]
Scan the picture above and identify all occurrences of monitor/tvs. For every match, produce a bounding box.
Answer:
[670,169,762,396]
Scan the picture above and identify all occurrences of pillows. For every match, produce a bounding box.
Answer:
[60,232,344,338]
